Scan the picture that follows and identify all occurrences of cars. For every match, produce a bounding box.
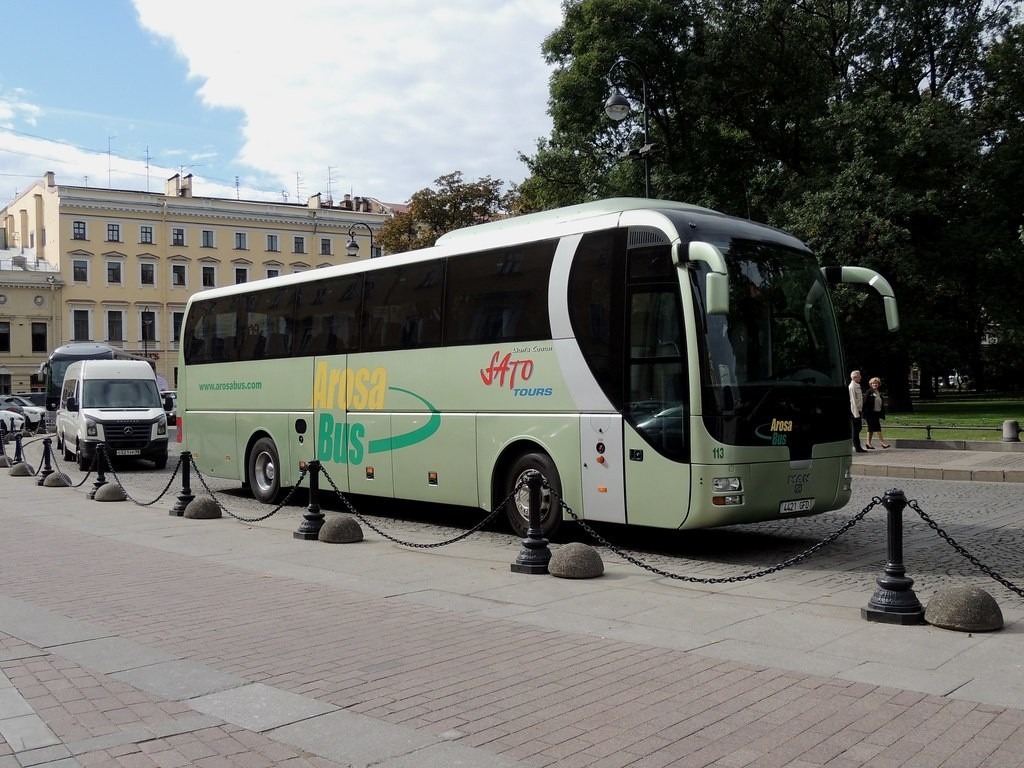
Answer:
[0,397,28,419]
[0,409,26,431]
[9,396,45,430]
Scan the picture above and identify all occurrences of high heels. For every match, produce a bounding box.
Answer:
[881,444,891,449]
[866,444,875,449]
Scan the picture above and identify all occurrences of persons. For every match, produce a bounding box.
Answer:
[848,370,868,452]
[864,377,891,449]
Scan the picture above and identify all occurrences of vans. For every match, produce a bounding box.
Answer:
[55,358,169,469]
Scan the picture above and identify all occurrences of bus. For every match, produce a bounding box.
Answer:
[37,342,158,432]
[176,196,901,526]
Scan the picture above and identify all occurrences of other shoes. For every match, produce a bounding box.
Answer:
[855,449,868,453]
